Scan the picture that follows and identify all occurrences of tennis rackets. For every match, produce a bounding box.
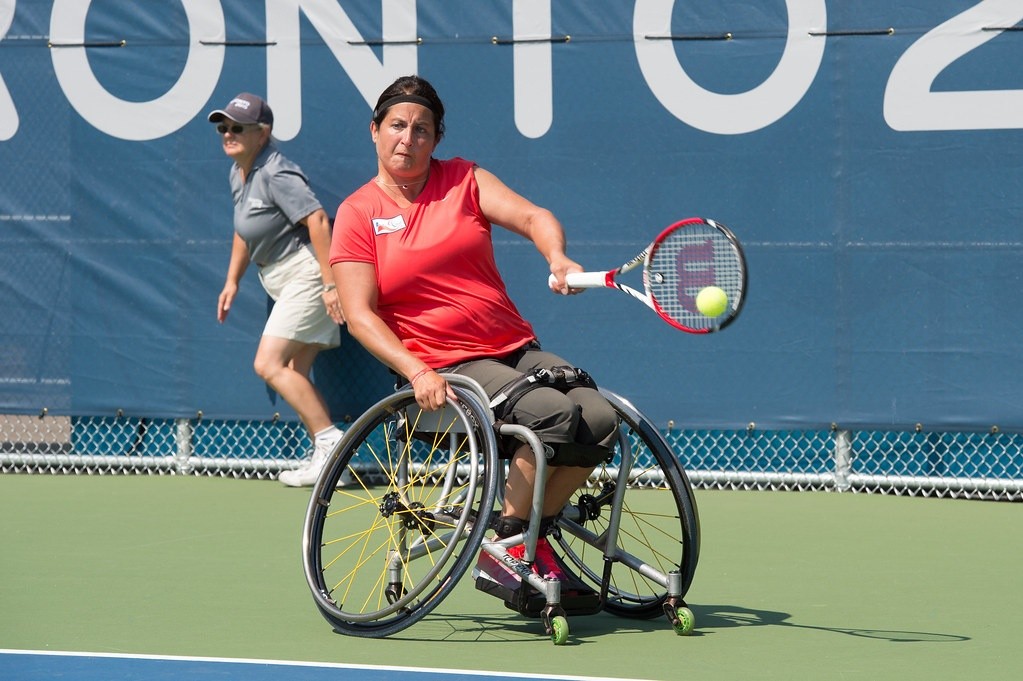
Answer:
[548,217,748,335]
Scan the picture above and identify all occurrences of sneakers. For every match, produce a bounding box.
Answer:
[508,537,570,592]
[301,430,346,483]
[278,465,355,491]
[469,539,546,601]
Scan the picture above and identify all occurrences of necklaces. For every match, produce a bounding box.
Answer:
[377,176,427,189]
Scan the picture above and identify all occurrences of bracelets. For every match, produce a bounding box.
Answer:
[323,284,335,292]
[412,367,433,386]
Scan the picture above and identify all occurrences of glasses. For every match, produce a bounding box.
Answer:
[216,124,244,135]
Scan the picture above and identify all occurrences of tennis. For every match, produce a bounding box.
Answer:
[695,286,728,318]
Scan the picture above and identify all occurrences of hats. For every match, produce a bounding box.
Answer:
[205,93,276,128]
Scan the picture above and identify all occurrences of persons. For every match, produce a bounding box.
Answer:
[329,77,618,597]
[205,92,353,487]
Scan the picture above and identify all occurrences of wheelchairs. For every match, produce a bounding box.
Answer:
[301,371,700,648]
